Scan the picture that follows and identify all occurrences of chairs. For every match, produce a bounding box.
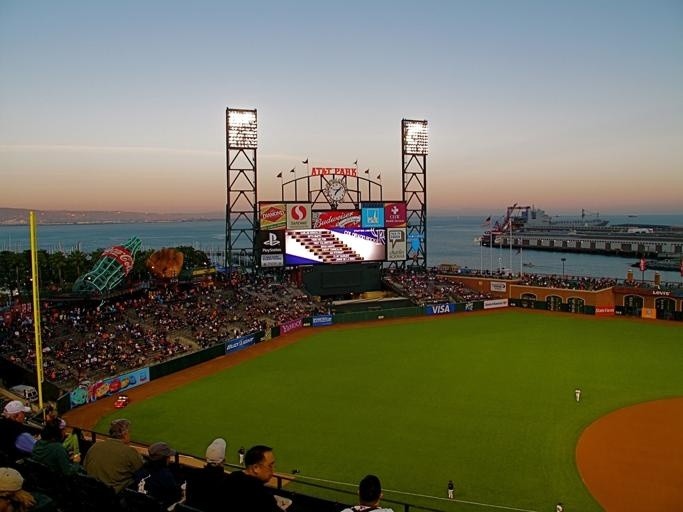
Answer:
[0,426,351,512]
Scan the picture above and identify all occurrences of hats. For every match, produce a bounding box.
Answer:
[3,400,31,416]
[0,467,25,492]
[148,441,176,458]
[206,438,227,467]
[359,475,382,506]
[110,419,132,437]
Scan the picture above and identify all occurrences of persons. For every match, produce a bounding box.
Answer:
[575,388,581,402]
[341,474,394,512]
[556,503,563,512]
[392,261,485,305]
[499,271,670,291]
[0,265,335,394]
[1,398,283,511]
[448,480,454,498]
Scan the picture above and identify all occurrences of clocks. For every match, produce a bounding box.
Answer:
[324,180,348,203]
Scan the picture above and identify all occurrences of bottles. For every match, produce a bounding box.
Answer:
[72,235,142,297]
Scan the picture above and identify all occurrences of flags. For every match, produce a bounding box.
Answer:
[276,172,282,178]
[352,161,357,164]
[376,175,379,179]
[484,217,491,226]
[301,160,308,164]
[289,168,294,173]
[364,169,368,174]
[492,222,502,235]
[502,220,510,231]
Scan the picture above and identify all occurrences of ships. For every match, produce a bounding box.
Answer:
[481,206,683,260]
